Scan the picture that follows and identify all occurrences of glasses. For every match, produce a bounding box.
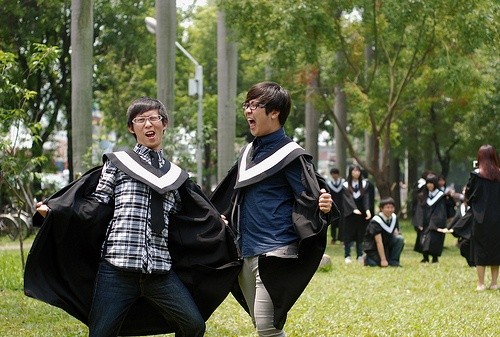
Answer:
[241,101,266,110]
[131,115,164,125]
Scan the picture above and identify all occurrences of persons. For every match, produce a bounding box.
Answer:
[357,167,375,220]
[325,167,347,245]
[23,99,243,337]
[435,184,478,266]
[411,172,458,263]
[361,197,405,267]
[463,144,500,291]
[209,81,342,337]
[340,164,372,264]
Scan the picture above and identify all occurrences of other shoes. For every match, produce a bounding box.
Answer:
[421,258,429,263]
[476,284,485,291]
[358,256,362,261]
[363,258,368,266]
[432,258,439,263]
[392,263,403,267]
[344,256,351,263]
[490,284,497,290]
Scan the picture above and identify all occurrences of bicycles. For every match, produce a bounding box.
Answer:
[0,205,33,241]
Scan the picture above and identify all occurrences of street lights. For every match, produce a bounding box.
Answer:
[143,14,203,186]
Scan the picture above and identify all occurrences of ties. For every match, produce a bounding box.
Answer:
[149,150,166,233]
[387,218,390,225]
[355,184,358,193]
